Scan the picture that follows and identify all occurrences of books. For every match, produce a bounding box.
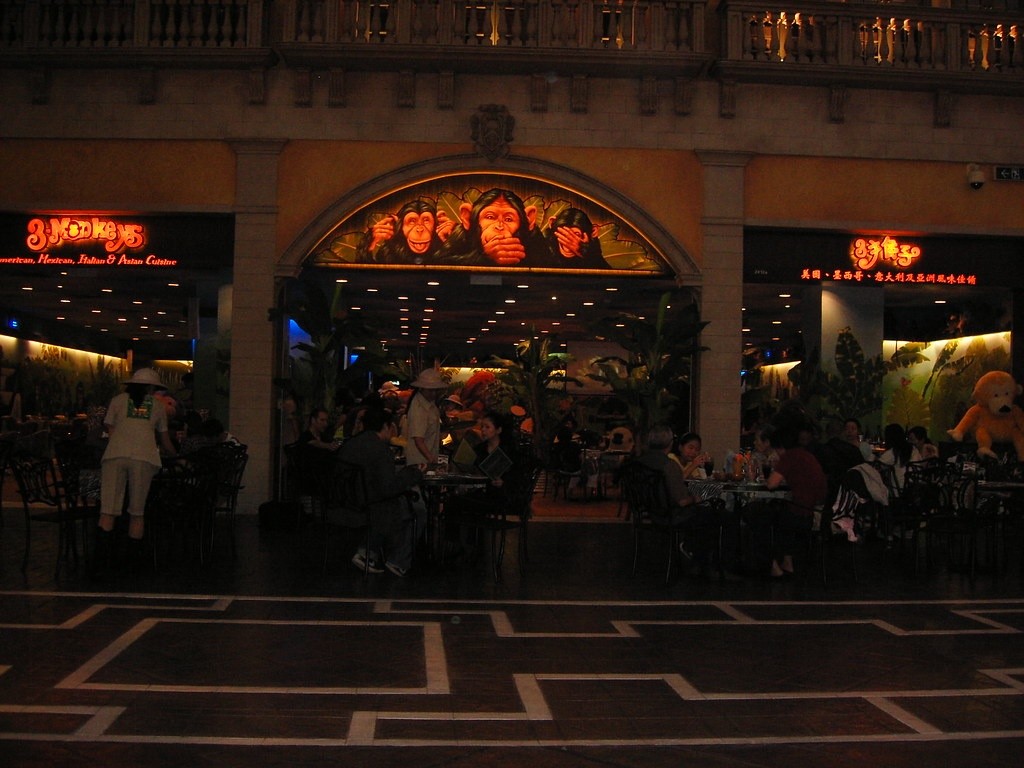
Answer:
[449,437,512,480]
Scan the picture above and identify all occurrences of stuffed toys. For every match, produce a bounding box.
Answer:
[946,371,1024,462]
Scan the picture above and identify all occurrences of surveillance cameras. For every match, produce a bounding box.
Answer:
[968,171,985,189]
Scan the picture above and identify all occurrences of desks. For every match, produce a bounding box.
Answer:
[951,480,1024,584]
[82,468,183,574]
[681,478,789,570]
[579,449,630,501]
[420,471,490,584]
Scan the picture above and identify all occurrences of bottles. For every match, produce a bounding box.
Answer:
[745,447,756,480]
[735,448,745,479]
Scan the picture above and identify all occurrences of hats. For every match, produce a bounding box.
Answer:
[119,368,168,392]
[409,369,449,389]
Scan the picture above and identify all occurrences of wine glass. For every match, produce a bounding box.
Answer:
[763,463,771,483]
[704,457,713,482]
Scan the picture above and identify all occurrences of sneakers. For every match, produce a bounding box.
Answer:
[352,553,385,573]
[383,560,408,579]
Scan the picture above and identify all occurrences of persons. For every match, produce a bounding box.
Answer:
[552,427,581,487]
[294,408,340,491]
[841,418,939,508]
[407,368,449,468]
[92,367,177,580]
[168,409,244,475]
[445,412,522,539]
[0,357,25,508]
[642,425,825,580]
[335,409,428,579]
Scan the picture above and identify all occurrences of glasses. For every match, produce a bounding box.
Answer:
[671,434,678,441]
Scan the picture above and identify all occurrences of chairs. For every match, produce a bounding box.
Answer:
[0,408,1024,613]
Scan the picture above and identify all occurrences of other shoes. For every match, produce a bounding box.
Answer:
[679,541,693,561]
[760,570,786,582]
[782,569,797,580]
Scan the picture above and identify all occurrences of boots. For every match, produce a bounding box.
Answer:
[123,535,146,583]
[94,524,122,577]
